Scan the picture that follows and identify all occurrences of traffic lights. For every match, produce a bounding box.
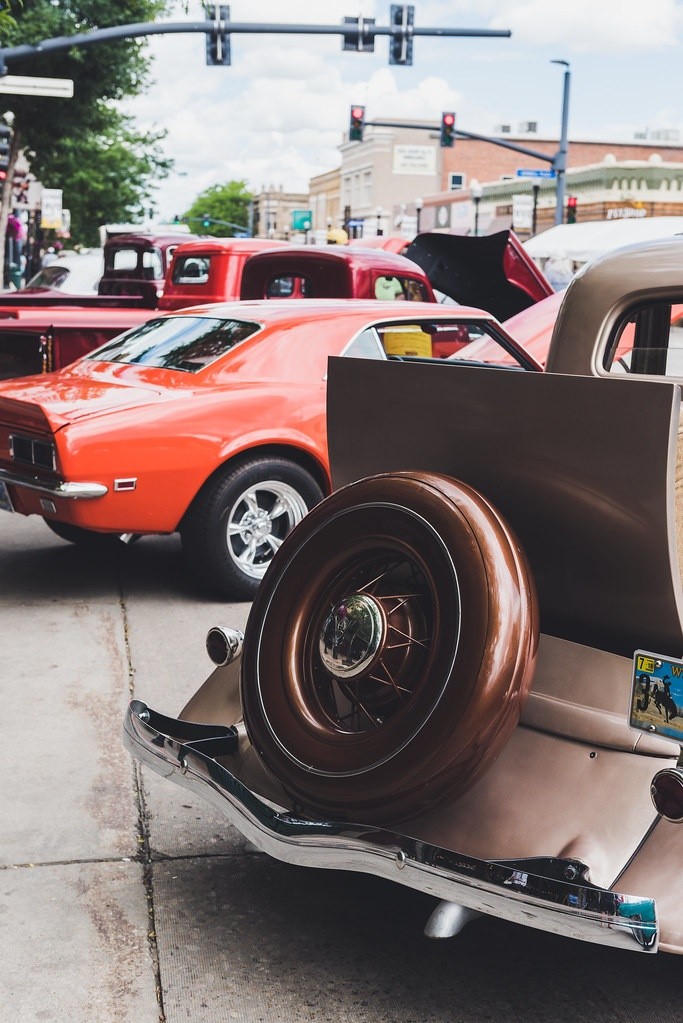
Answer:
[565,197,577,224]
[348,105,365,140]
[441,111,456,146]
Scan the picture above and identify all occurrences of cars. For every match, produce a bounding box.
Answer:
[119,229,683,972]
[1,217,683,605]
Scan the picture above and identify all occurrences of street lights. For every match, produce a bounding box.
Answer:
[472,183,483,237]
[549,58,570,227]
[413,197,423,236]
